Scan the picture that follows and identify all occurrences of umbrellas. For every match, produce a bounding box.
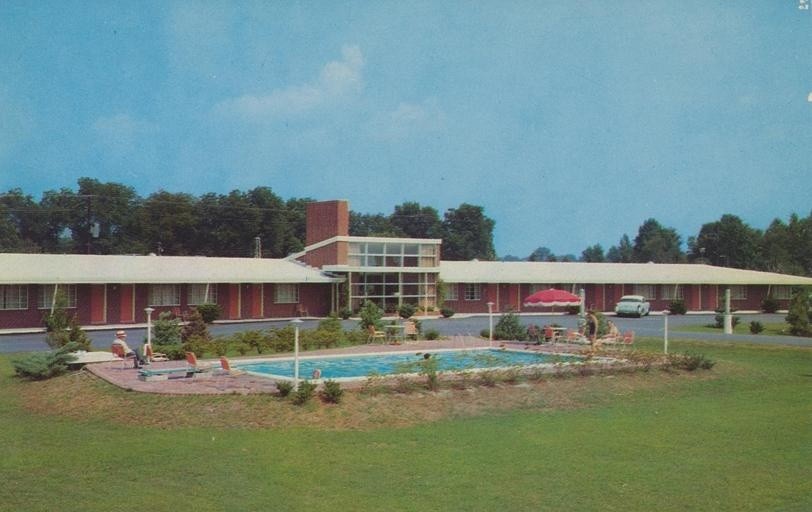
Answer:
[523,288,582,326]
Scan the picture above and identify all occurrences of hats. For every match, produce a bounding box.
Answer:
[114,330,127,337]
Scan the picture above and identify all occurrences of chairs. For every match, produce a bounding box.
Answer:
[112,343,169,369]
[306,368,321,382]
[368,321,419,344]
[526,322,635,349]
[295,303,308,316]
[184,350,246,385]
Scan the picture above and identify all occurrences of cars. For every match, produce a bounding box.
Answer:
[615,294,651,318]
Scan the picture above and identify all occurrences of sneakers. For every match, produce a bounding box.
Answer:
[140,361,149,365]
[133,365,142,369]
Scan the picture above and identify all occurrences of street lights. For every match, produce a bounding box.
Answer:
[144,307,155,365]
[662,310,671,355]
[485,301,494,348]
[290,317,305,393]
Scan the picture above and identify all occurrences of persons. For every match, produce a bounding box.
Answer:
[424,353,432,360]
[111,330,150,368]
